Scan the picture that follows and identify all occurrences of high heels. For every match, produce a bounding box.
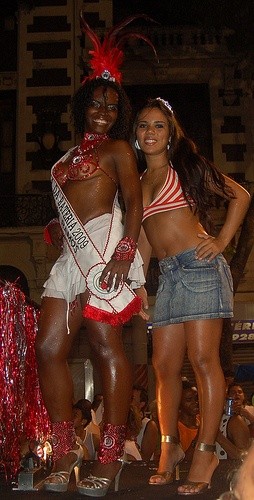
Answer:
[76,450,127,497]
[177,441,219,496]
[44,441,84,492]
[148,435,185,485]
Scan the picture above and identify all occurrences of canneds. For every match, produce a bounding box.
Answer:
[226,397,235,416]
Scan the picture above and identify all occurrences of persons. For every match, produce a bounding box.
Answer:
[133,97,250,497]
[70,369,254,462]
[35,69,142,497]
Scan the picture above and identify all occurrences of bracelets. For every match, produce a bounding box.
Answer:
[42,217,62,245]
[109,236,137,264]
